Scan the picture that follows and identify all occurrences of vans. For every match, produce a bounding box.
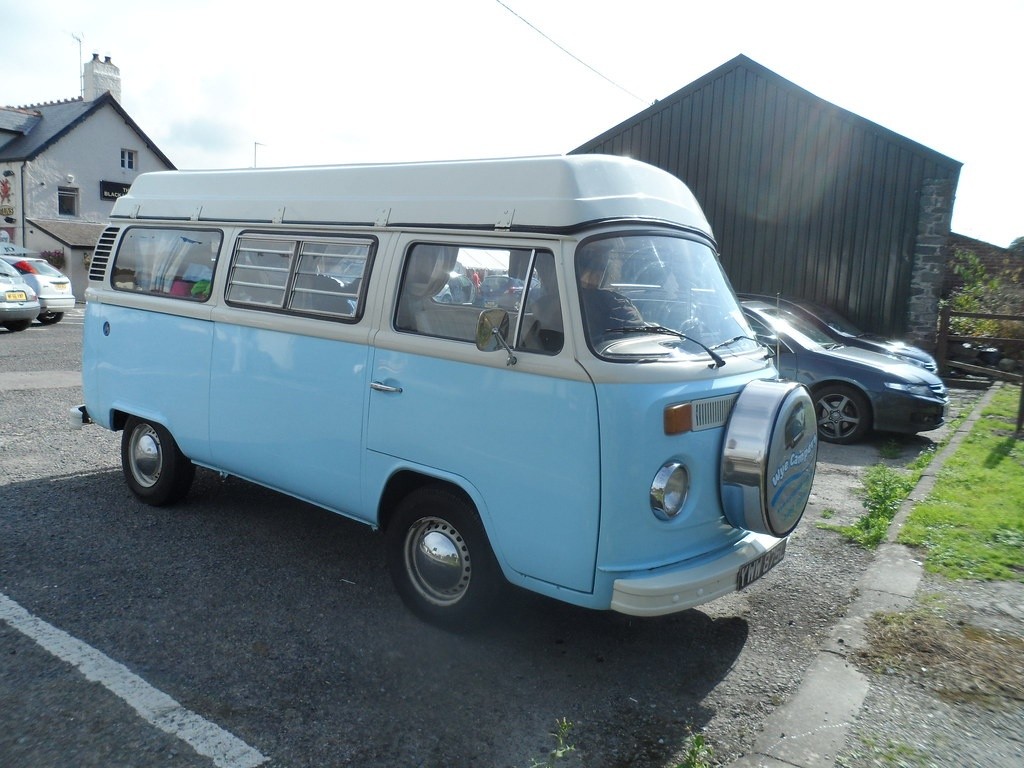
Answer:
[66,149,819,631]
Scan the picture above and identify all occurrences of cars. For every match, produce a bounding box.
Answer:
[729,297,951,443]
[772,292,939,377]
[0,255,78,325]
[0,258,41,333]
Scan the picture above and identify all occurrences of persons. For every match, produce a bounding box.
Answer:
[529,240,645,352]
